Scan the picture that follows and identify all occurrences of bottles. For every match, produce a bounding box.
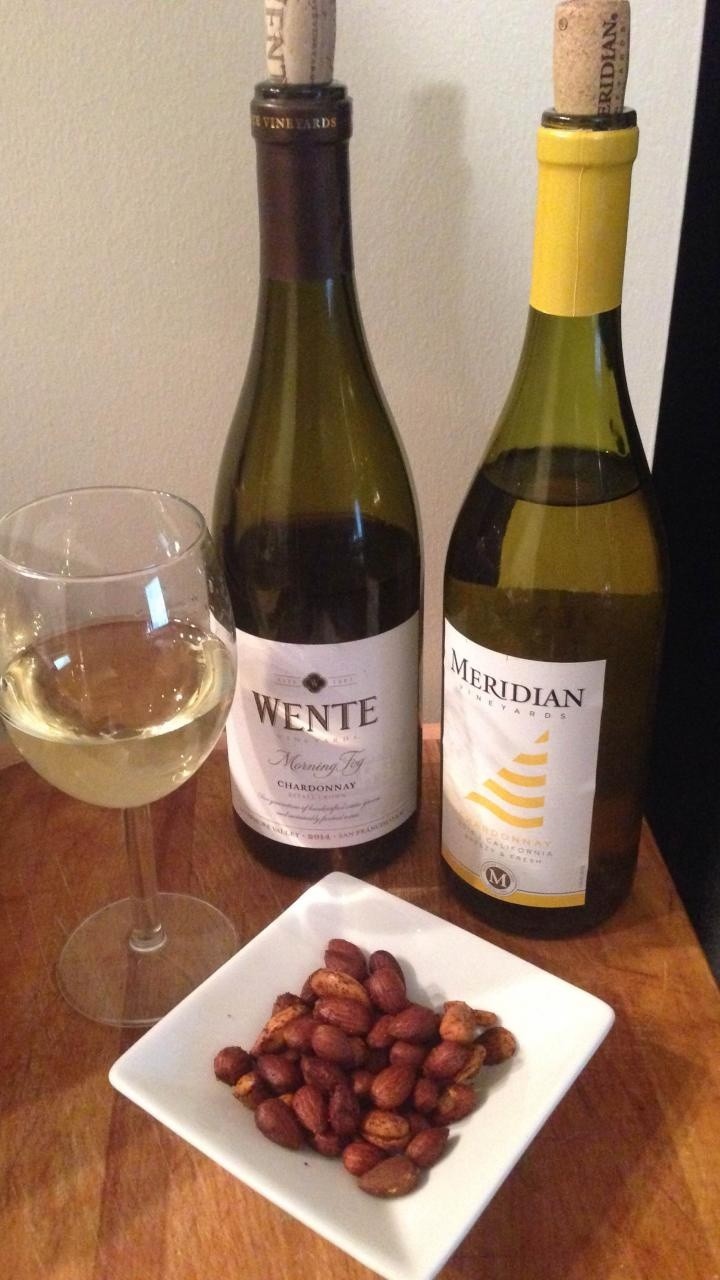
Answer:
[216,0,420,876]
[437,0,668,940]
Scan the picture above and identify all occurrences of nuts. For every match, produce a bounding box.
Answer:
[213,939,515,1201]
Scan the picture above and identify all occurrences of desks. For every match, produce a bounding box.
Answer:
[0,723,720,1280]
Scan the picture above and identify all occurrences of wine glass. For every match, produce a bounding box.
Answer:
[0,482,237,1025]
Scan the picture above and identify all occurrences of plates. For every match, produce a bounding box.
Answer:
[109,870,617,1280]
